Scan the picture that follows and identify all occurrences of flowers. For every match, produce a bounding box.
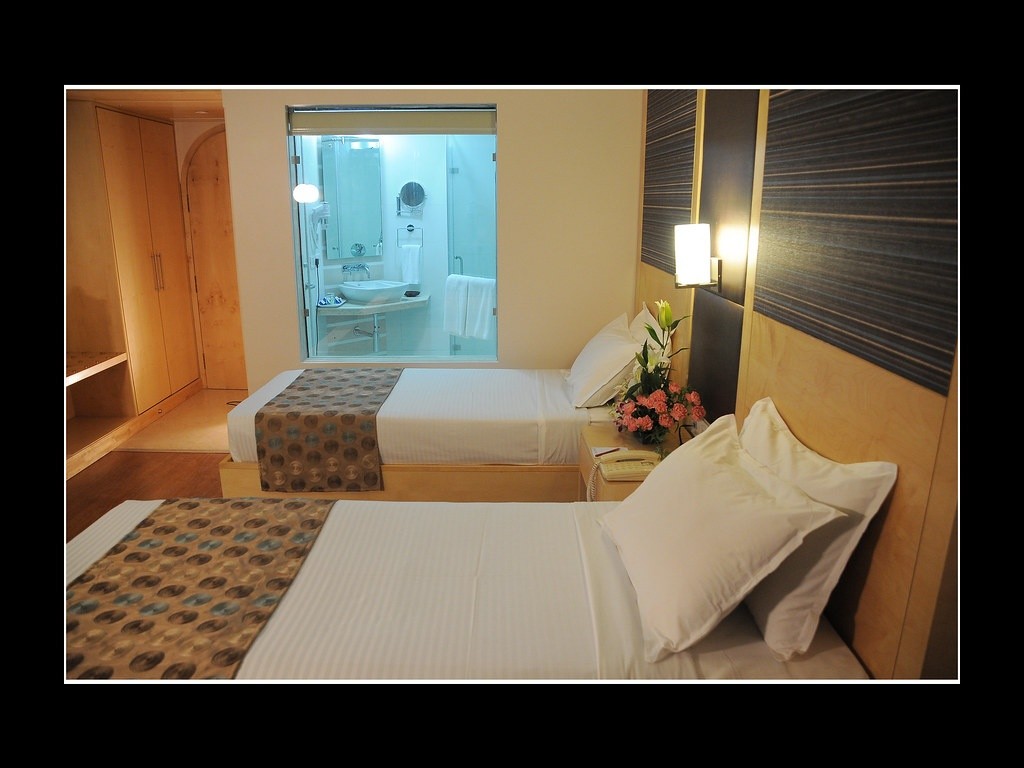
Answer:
[606,300,707,459]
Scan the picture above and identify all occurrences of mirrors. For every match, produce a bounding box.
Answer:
[321,139,384,259]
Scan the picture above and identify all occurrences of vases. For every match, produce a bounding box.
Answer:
[631,411,666,445]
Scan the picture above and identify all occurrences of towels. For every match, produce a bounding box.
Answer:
[375,243,383,256]
[443,274,497,342]
[401,245,422,284]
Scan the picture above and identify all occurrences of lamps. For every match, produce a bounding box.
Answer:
[674,223,722,294]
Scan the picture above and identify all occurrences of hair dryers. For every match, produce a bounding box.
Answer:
[311,201,330,231]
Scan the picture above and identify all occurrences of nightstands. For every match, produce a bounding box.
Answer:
[579,425,692,501]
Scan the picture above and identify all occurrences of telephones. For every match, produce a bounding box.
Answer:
[598,449,661,482]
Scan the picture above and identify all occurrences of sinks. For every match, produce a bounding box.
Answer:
[338,280,409,302]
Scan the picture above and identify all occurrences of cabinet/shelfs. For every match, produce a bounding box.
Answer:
[66,100,203,481]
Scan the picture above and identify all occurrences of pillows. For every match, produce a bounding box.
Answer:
[561,313,644,411]
[739,397,898,662]
[596,414,850,664]
[614,301,673,406]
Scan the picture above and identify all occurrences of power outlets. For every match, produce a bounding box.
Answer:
[310,254,320,270]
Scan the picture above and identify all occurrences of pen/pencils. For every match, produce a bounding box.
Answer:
[595,447,619,458]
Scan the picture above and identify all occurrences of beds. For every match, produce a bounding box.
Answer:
[220,368,623,500]
[66,499,874,680]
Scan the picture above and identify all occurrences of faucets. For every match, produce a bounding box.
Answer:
[363,264,371,278]
